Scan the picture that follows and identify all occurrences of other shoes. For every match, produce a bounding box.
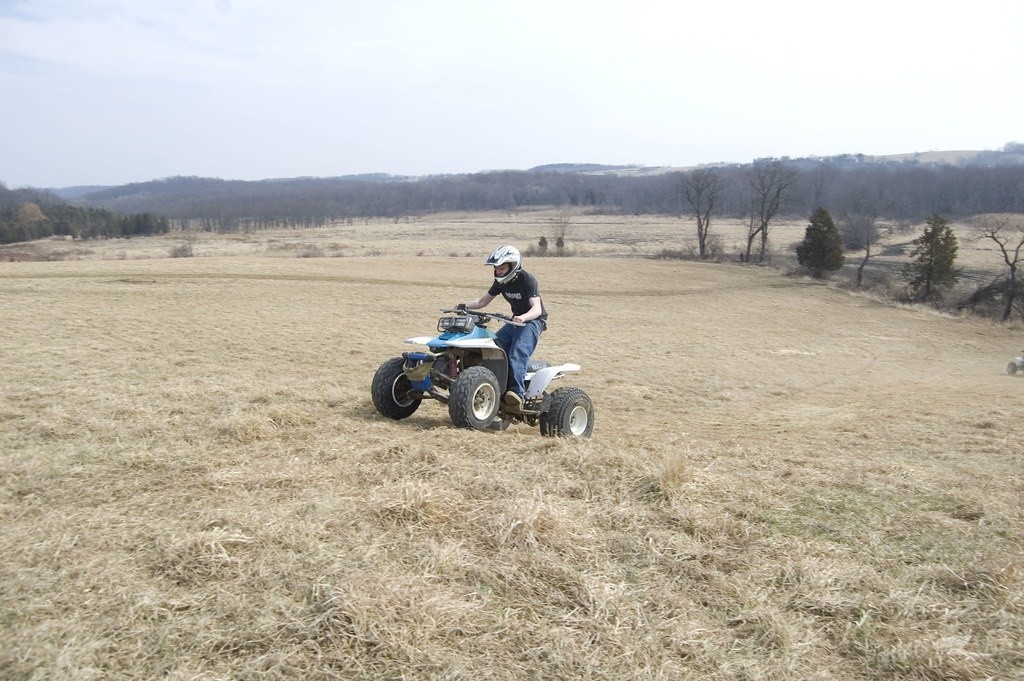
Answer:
[504,390,523,410]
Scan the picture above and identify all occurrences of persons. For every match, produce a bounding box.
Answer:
[454,245,548,415]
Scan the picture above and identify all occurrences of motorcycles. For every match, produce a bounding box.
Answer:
[371,303,596,440]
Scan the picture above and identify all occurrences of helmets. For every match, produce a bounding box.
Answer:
[484,245,523,285]
